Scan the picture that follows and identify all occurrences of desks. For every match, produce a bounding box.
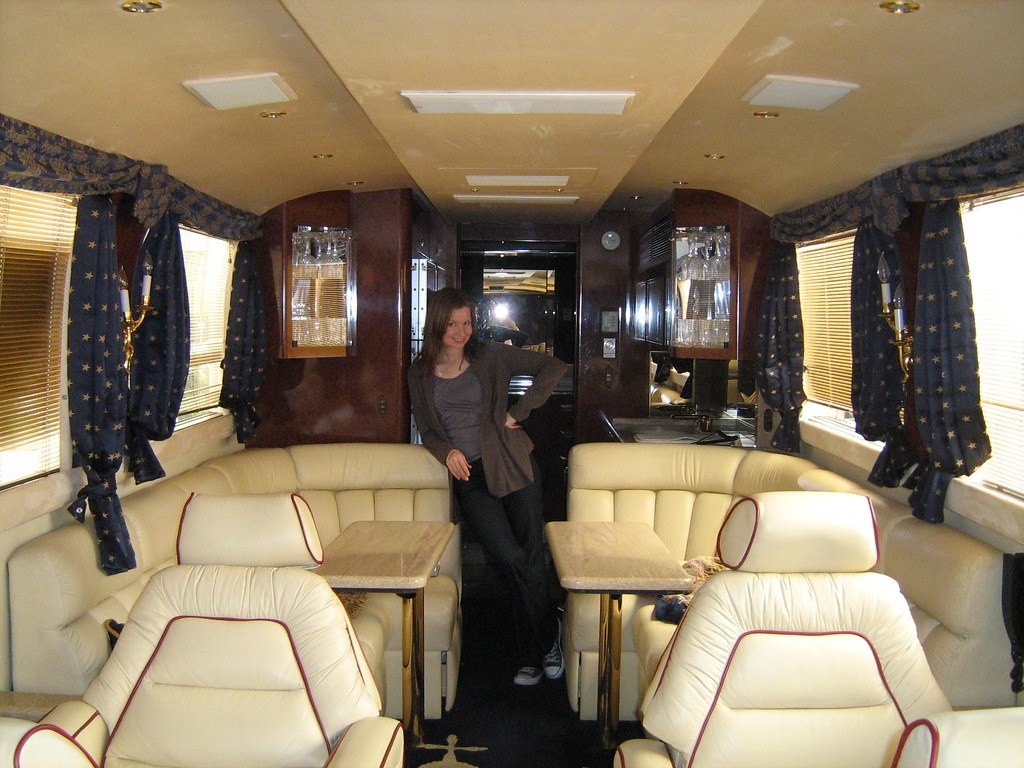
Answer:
[543,517,694,758]
[600,408,755,450]
[313,520,454,757]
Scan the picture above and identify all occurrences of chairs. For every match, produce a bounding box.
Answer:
[12,490,404,768]
[615,491,954,768]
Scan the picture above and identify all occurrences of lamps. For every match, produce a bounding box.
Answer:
[875,251,916,427]
[117,250,156,373]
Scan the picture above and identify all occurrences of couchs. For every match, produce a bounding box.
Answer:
[5,442,466,730]
[562,443,1020,725]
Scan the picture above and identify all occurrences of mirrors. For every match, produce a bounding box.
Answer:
[482,268,555,357]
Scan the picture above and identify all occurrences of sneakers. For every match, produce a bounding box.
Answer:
[543,617,565,680]
[514,666,543,685]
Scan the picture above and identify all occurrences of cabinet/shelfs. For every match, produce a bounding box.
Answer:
[408,200,446,444]
[632,261,667,346]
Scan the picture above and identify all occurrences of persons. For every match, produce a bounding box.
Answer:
[405,286,570,686]
